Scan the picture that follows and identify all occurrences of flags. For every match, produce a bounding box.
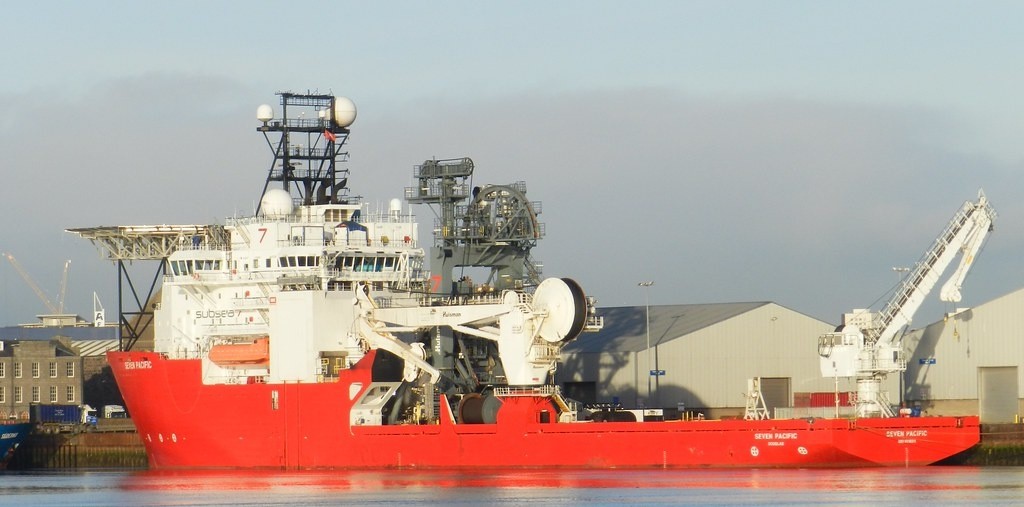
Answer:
[324,129,336,142]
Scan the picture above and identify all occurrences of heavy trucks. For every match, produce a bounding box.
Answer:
[29,402,98,426]
[101,404,127,418]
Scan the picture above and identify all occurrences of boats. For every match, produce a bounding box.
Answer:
[104,88,1002,474]
[0,418,40,473]
